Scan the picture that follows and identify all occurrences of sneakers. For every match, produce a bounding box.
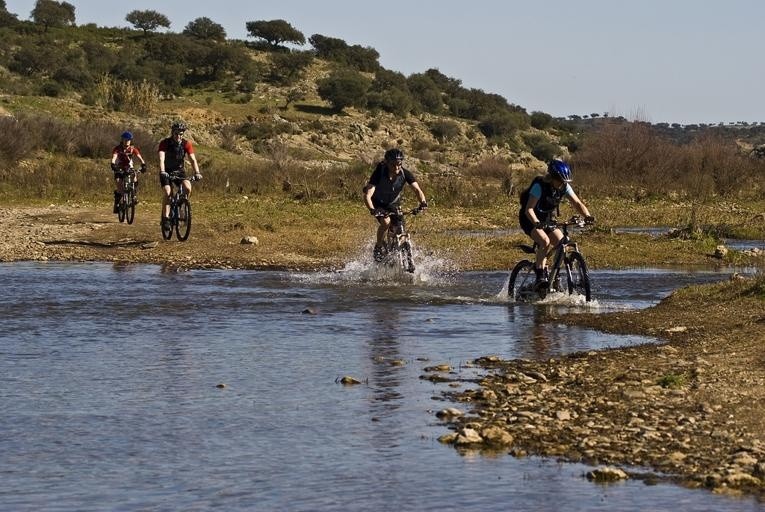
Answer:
[113,204,121,214]
[163,218,173,232]
[372,245,384,263]
[536,268,550,288]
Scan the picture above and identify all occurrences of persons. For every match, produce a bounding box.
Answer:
[519,160,594,292]
[363,150,427,261]
[158,123,203,230]
[111,133,145,208]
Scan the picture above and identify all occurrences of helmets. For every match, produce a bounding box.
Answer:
[384,149,405,161]
[120,131,134,140]
[172,122,187,132]
[546,161,574,182]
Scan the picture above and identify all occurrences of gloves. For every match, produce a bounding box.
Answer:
[370,207,388,218]
[538,222,550,229]
[113,166,124,174]
[139,165,146,174]
[161,172,170,178]
[195,173,203,181]
[418,203,428,212]
[585,218,596,226]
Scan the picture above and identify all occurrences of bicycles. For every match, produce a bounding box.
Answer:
[373,203,425,276]
[110,168,144,224]
[159,171,203,244]
[506,216,595,305]
